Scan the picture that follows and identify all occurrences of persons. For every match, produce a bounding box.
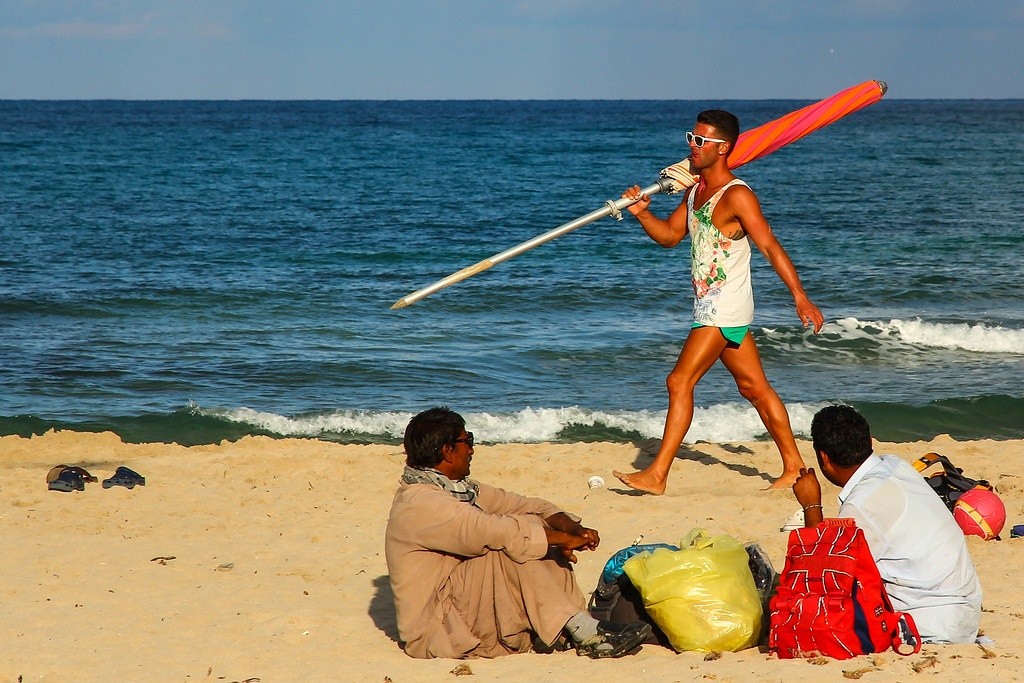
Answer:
[792,404,983,646]
[383,405,653,660]
[612,109,824,497]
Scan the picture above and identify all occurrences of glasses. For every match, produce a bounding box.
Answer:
[685,132,726,147]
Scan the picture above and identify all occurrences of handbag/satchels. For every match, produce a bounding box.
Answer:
[588,540,684,644]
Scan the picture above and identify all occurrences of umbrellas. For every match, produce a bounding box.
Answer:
[390,81,890,310]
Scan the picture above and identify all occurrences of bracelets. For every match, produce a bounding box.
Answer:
[802,504,823,512]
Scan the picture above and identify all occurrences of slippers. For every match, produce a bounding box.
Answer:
[102,466,146,489]
[48,466,98,492]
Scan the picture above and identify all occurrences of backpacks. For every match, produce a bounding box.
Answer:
[770,517,921,660]
[912,453,993,515]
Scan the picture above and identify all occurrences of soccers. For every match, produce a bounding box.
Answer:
[953,488,1007,541]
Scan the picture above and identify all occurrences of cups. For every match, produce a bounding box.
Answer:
[588,476,605,490]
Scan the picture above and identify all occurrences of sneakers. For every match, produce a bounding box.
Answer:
[574,621,652,658]
